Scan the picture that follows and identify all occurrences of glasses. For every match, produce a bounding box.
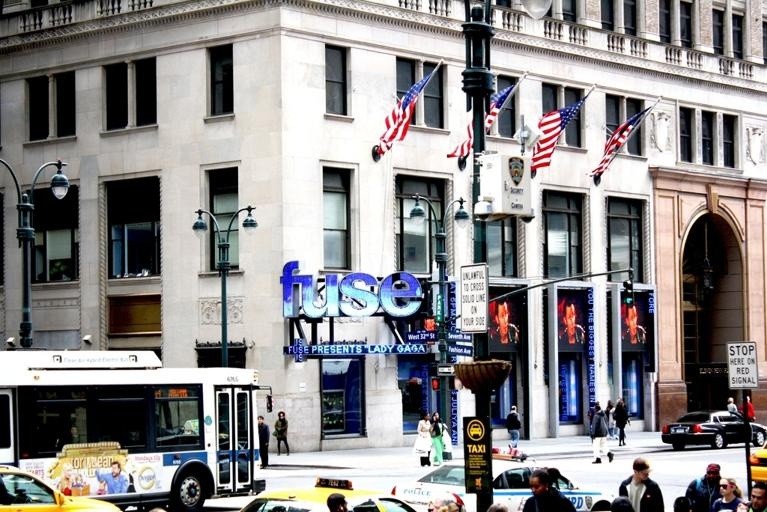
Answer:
[719,485,727,489]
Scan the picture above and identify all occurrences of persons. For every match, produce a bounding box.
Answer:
[738,396,757,421]
[275,410,293,451]
[561,298,589,346]
[489,299,520,344]
[413,404,578,512]
[260,412,270,468]
[673,463,767,512]
[94,461,130,494]
[727,397,738,412]
[621,300,648,344]
[326,493,353,511]
[587,397,663,512]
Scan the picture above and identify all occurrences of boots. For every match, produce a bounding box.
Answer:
[607,452,613,462]
[592,458,601,463]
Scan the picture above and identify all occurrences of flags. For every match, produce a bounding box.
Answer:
[445,82,516,158]
[591,105,652,177]
[376,64,440,155]
[530,96,588,170]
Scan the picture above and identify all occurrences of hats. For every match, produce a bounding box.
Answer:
[633,458,653,474]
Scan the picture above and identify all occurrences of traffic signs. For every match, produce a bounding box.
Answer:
[446,331,473,343]
[447,344,475,357]
[436,292,443,321]
[405,331,439,343]
[436,363,457,377]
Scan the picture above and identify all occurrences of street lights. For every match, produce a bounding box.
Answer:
[191,206,260,366]
[0,156,70,348]
[409,191,470,460]
[460,0,554,512]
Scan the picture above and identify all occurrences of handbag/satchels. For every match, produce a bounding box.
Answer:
[413,433,429,458]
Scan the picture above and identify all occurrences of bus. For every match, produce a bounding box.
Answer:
[0,348,275,512]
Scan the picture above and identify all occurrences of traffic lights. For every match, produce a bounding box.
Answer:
[421,317,439,333]
[431,377,442,392]
[621,278,635,309]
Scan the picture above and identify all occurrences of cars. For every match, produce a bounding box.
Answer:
[750,437,767,487]
[661,408,767,451]
[0,463,122,512]
[389,446,613,512]
[234,475,420,512]
